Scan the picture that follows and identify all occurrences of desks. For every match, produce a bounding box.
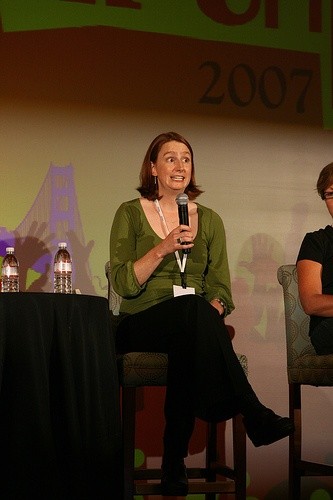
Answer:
[0,292,136,500]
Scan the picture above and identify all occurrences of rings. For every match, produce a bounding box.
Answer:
[176,237,182,244]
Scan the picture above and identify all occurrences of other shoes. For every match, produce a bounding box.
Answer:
[242,408,295,448]
[160,459,190,496]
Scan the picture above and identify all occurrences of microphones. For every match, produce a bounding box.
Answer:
[176,192,191,254]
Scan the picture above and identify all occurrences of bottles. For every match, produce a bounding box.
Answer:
[54,242,73,293]
[1,247,20,292]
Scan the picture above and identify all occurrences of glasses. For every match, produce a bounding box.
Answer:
[323,191,333,200]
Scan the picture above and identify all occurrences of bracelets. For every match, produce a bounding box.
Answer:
[213,298,226,309]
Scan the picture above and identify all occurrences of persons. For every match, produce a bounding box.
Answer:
[296,162,333,355]
[109,132,296,496]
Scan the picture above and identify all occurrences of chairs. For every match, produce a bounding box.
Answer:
[276,263,333,500]
[104,260,249,500]
